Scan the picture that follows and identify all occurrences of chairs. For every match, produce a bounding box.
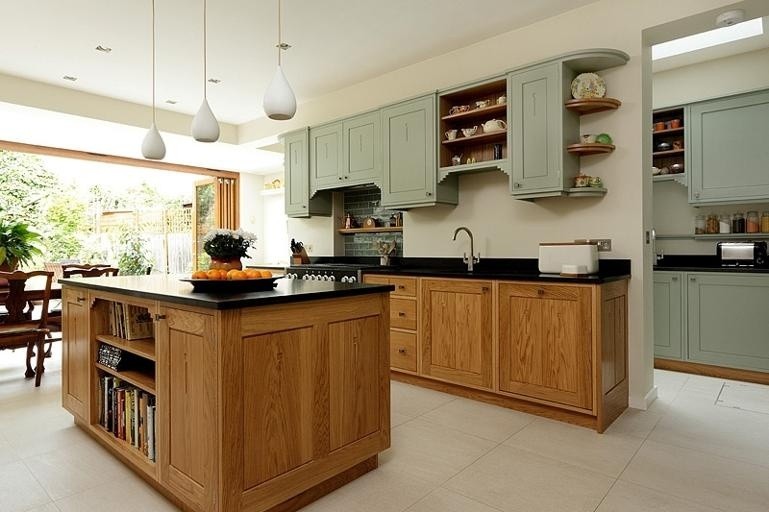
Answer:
[35,268,120,373]
[61,264,110,278]
[0,269,54,387]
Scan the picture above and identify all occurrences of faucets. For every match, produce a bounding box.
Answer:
[452,226,481,271]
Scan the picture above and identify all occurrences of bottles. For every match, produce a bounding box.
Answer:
[344,211,353,229]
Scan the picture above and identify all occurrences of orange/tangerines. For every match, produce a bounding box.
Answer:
[192,268,272,280]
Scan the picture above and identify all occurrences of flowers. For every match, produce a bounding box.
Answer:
[202,228,258,260]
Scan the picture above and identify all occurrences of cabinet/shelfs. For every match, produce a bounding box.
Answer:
[652,105,689,188]
[419,277,494,406]
[508,47,629,201]
[278,126,332,220]
[331,189,404,256]
[436,72,508,185]
[88,286,158,496]
[154,287,391,512]
[380,89,458,211]
[688,89,769,208]
[308,106,381,201]
[362,273,418,387]
[60,285,87,436]
[653,270,769,385]
[494,281,628,434]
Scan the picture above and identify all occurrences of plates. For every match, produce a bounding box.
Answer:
[179,274,286,287]
[571,73,605,98]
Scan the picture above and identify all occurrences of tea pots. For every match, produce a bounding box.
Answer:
[481,119,507,133]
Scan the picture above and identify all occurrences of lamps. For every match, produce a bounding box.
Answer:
[142,0,166,161]
[189,1,220,143]
[262,1,297,120]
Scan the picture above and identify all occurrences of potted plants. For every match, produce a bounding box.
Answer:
[0,217,44,274]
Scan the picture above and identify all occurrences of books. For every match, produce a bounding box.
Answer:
[99,374,155,461]
[107,301,152,340]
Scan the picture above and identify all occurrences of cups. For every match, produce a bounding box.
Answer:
[476,100,490,109]
[444,130,457,140]
[461,126,478,136]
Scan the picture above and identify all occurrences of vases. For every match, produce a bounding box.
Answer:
[208,257,243,271]
